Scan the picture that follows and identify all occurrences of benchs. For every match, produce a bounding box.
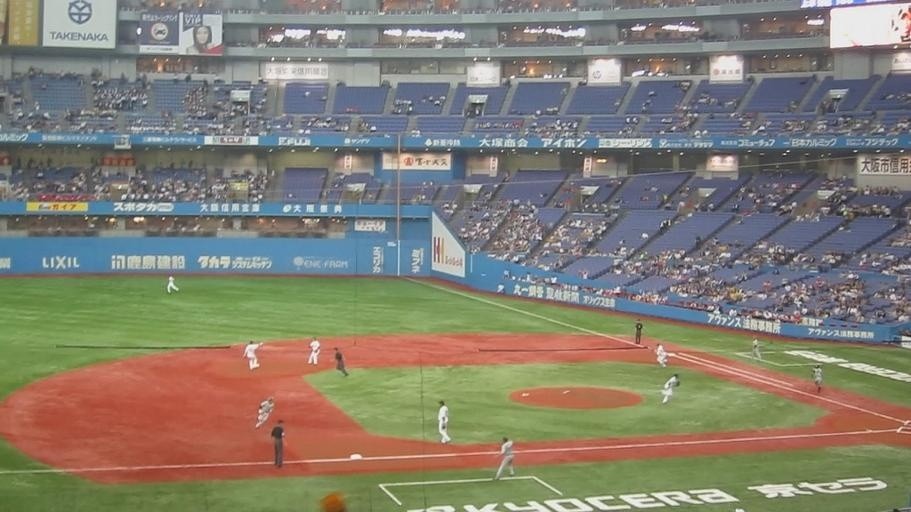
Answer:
[443,72,911,326]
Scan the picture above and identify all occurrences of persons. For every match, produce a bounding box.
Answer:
[2,65,911,139]
[660,374,679,404]
[0,158,360,232]
[635,320,642,344]
[255,397,274,429]
[334,347,348,377]
[810,364,823,393]
[751,336,761,360]
[307,337,321,365]
[417,168,911,324]
[654,344,666,367]
[270,419,285,469]
[167,275,179,294]
[493,437,515,480]
[437,400,451,444]
[187,25,221,55]
[242,340,264,370]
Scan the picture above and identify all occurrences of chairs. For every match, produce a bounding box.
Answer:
[0,71,443,207]
